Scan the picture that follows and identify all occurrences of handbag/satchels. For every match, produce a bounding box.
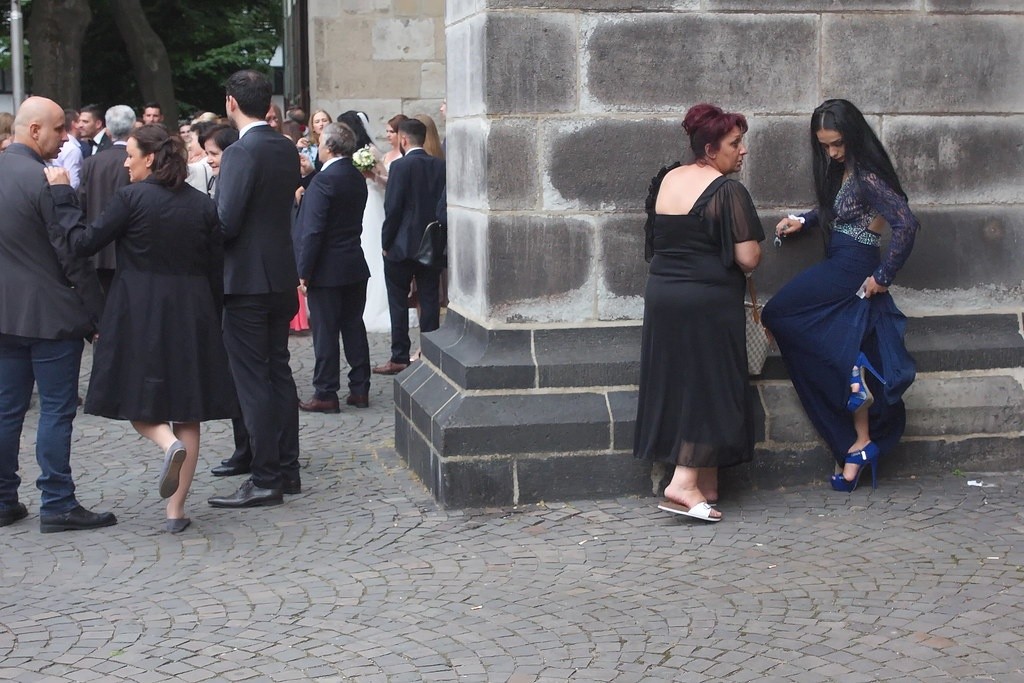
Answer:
[745,271,776,375]
[415,218,449,269]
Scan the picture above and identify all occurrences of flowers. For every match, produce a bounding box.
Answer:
[351,148,377,174]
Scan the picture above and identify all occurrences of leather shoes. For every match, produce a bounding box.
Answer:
[346,394,368,409]
[39,505,117,533]
[286,473,302,495]
[211,463,251,476]
[0,501,28,527]
[298,395,340,414]
[372,360,407,374]
[206,479,284,508]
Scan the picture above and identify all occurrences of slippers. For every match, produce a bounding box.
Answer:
[658,501,721,523]
[706,500,720,508]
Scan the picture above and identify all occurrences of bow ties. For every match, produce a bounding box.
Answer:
[87,138,98,147]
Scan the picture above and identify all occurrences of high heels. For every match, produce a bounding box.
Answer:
[830,442,880,493]
[846,351,887,414]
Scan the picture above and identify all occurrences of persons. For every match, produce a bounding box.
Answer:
[0,96,119,533]
[0,99,453,475]
[761,98,921,492]
[633,104,765,522]
[44,123,238,535]
[207,69,302,509]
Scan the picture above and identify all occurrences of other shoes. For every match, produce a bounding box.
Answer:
[158,441,185,498]
[164,518,191,533]
